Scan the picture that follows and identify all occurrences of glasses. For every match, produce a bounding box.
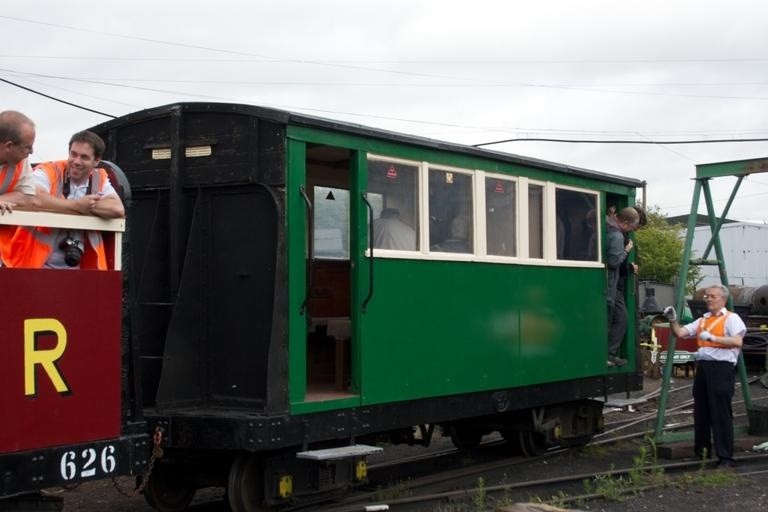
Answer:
[703,294,718,300]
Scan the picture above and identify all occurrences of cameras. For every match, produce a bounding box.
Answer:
[59,238,85,267]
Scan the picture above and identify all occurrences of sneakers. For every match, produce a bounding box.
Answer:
[682,456,735,468]
[608,357,628,367]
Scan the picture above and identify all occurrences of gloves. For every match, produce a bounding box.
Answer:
[662,306,677,321]
[700,331,715,342]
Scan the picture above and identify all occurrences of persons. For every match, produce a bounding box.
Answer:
[369,208,418,249]
[0,110,126,270]
[430,214,471,254]
[606,206,647,367]
[662,285,747,470]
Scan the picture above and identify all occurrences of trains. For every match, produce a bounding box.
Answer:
[3,99,651,512]
